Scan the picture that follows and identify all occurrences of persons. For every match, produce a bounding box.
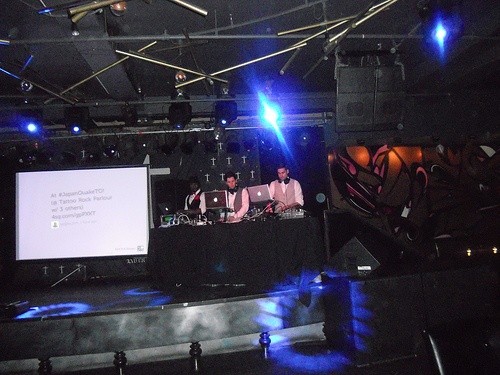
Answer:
[184,176,207,215]
[219,170,250,222]
[268,164,305,214]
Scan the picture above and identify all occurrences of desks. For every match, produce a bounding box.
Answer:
[148,219,323,289]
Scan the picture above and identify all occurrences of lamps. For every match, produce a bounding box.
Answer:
[110,1,127,16]
[175,41,187,83]
[16,89,238,133]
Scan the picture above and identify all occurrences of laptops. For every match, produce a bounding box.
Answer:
[204,190,229,208]
[248,184,274,202]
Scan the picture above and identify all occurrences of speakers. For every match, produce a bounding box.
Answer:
[330,236,384,280]
[336,64,410,131]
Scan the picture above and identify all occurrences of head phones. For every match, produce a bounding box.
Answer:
[227,186,238,191]
[279,176,290,183]
